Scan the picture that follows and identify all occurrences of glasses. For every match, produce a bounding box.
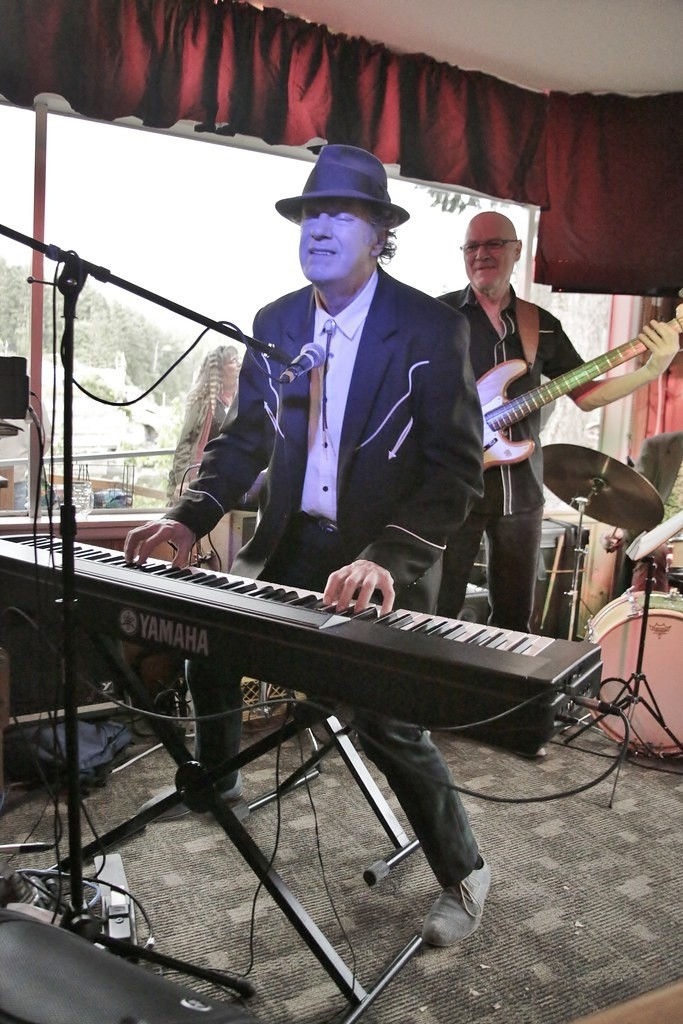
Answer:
[460,238,518,254]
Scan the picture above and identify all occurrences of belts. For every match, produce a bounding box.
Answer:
[290,511,337,537]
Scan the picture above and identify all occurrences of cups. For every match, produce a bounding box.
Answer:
[58,480,94,520]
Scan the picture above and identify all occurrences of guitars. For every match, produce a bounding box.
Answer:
[476,301,683,475]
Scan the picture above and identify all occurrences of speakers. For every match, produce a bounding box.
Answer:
[456,582,490,625]
[0,908,269,1024]
[0,607,134,729]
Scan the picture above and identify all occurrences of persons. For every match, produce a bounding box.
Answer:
[124,143,492,946]
[0,402,52,512]
[165,346,240,510]
[433,212,680,760]
[600,430,683,592]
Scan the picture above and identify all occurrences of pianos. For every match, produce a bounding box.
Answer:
[0,532,605,756]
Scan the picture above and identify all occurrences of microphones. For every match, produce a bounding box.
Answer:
[280,342,326,383]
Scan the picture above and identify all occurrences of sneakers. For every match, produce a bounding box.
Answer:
[509,747,547,758]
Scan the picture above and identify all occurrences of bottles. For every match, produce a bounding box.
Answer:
[25,465,48,521]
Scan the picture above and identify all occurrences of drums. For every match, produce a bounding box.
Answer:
[583,589,683,757]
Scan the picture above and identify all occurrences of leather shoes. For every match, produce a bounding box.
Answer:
[422,852,491,947]
[136,770,243,822]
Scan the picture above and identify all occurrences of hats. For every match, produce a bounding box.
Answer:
[275,144,410,230]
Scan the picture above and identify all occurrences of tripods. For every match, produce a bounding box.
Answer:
[565,511,683,810]
[0,223,295,999]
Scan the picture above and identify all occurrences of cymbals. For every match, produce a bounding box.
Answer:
[542,442,664,533]
